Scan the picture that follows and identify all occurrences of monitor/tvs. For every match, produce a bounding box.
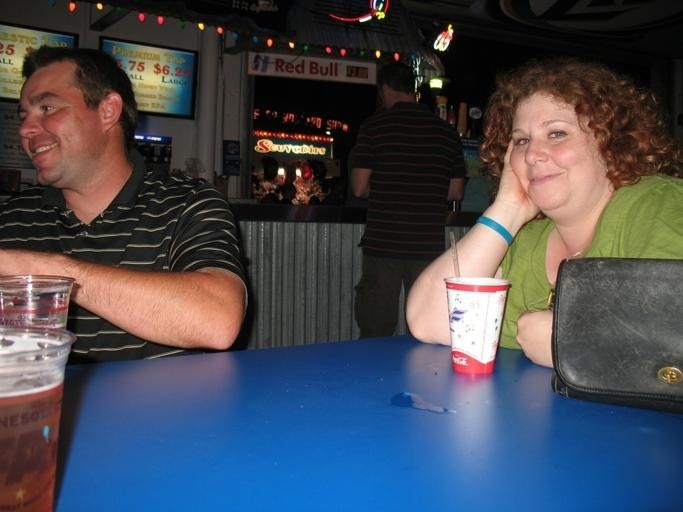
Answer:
[250,101,348,206]
[459,136,486,177]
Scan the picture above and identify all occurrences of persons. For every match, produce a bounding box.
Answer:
[0,44,254,362]
[347,58,467,341]
[403,54,682,369]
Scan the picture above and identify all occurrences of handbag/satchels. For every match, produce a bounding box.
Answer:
[552,257,683,413]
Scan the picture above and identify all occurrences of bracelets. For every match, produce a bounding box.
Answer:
[475,215,514,246]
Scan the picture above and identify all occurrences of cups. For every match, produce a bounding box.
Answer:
[0,274,76,333]
[0,322,78,512]
[443,275,511,376]
